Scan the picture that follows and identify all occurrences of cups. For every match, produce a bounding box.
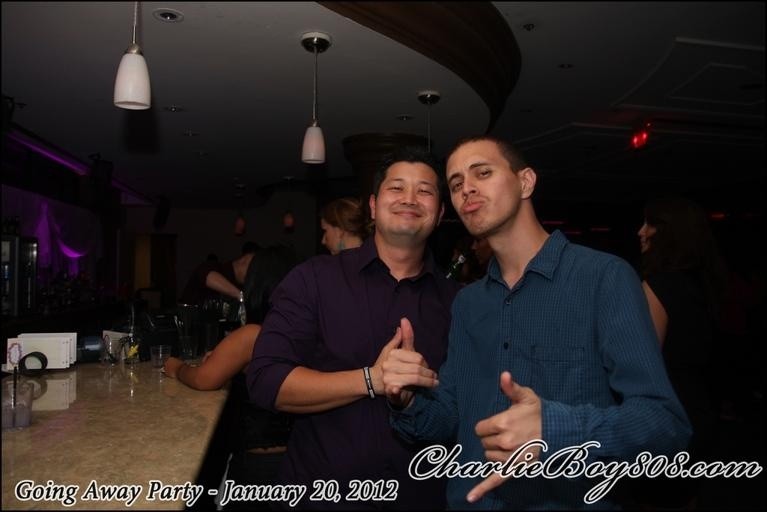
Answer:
[0,382,34,428]
[149,344,172,373]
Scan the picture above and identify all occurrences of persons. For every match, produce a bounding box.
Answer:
[233,145,465,510]
[161,192,764,483]
[371,129,697,512]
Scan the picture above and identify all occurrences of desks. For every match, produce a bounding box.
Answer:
[0,359,232,511]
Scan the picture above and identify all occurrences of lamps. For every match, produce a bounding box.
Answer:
[300,31,333,164]
[113,1,152,110]
